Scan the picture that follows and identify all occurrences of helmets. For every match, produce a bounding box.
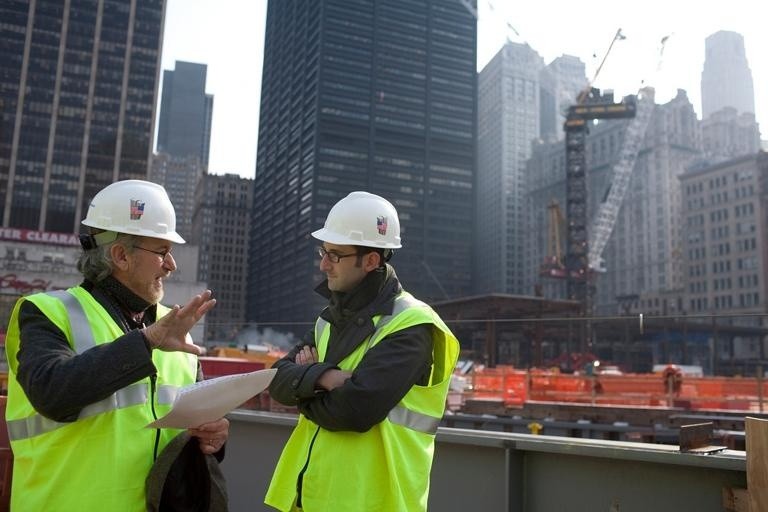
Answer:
[81,179,186,244]
[311,191,403,248]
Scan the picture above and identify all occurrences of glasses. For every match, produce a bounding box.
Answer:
[319,247,357,262]
[134,246,173,261]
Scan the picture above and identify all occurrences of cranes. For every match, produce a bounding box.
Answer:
[558,30,689,369]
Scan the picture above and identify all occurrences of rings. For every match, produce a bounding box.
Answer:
[209,439,214,447]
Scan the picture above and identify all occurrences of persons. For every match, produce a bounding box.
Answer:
[5,180,230,511]
[263,191,461,512]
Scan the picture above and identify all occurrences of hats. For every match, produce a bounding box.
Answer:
[145,430,229,512]
